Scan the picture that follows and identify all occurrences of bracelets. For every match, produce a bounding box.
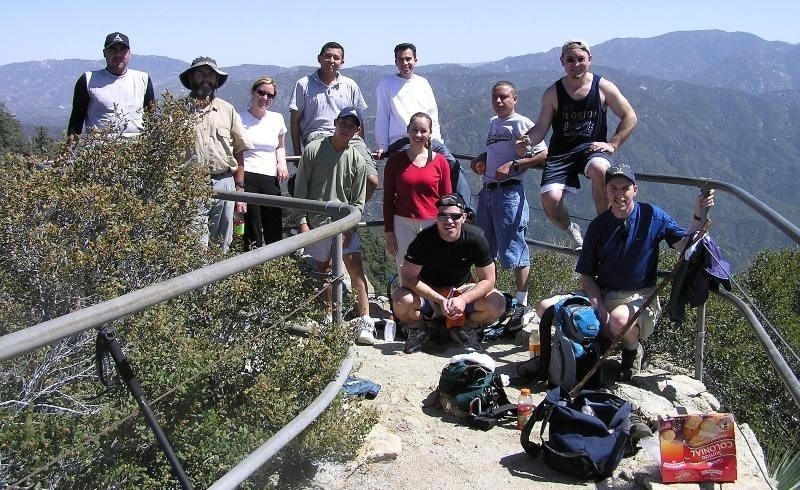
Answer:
[694,213,701,221]
[235,181,248,189]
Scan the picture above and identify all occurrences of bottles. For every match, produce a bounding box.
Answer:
[232,208,246,237]
[518,388,532,430]
[528,329,540,360]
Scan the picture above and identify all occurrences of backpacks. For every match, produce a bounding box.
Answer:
[432,354,517,434]
[537,294,606,391]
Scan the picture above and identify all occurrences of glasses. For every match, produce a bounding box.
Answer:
[437,211,462,221]
[254,88,274,99]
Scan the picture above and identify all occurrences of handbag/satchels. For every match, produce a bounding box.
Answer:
[521,387,631,479]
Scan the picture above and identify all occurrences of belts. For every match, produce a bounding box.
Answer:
[482,179,522,192]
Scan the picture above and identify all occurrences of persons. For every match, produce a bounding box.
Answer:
[536,162,715,381]
[167,57,257,295]
[512,40,638,252]
[470,79,546,331]
[292,106,376,345]
[392,194,507,353]
[374,43,476,219]
[55,32,155,163]
[287,42,377,204]
[383,112,452,287]
[237,75,287,252]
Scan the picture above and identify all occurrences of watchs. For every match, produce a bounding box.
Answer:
[512,158,521,173]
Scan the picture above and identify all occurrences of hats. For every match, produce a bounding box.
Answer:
[179,56,228,91]
[605,163,637,187]
[434,194,467,208]
[105,32,130,50]
[339,106,364,125]
[560,38,591,56]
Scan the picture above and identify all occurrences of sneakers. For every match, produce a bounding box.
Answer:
[621,343,644,378]
[572,223,584,253]
[449,326,484,352]
[405,330,430,354]
[358,317,377,344]
[507,304,535,330]
